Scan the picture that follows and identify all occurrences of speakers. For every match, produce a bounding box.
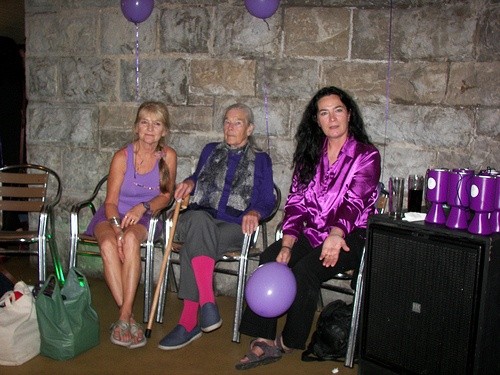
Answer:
[360,212,500,375]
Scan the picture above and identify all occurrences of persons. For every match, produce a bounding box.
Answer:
[157,104,273,351]
[0,36,29,248]
[83,102,177,350]
[236,85,381,370]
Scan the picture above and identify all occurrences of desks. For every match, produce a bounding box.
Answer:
[357,205,500,375]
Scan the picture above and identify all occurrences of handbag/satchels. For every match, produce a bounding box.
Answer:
[0,281,41,365]
[36,268,100,361]
[310,300,356,358]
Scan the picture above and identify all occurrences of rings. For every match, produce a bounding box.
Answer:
[130,219,135,222]
[125,215,128,219]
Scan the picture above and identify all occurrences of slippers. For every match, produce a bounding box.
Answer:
[128,320,146,348]
[110,319,133,345]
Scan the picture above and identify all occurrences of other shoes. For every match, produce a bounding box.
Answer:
[159,324,203,350]
[198,297,222,332]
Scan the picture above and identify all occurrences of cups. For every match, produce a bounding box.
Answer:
[408,174,424,213]
[388,177,405,219]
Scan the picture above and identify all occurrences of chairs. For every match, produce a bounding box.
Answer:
[70,173,179,322]
[275,187,390,369]
[0,164,66,295]
[157,171,281,344]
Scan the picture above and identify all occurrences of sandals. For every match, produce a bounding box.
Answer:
[235,339,282,369]
[274,334,294,352]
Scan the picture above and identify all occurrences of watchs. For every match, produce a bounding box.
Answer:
[140,201,150,213]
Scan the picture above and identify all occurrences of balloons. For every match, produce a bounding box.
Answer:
[245,262,297,319]
[121,0,154,24]
[245,0,280,19]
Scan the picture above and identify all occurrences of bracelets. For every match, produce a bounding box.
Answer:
[282,245,293,250]
[110,216,121,227]
